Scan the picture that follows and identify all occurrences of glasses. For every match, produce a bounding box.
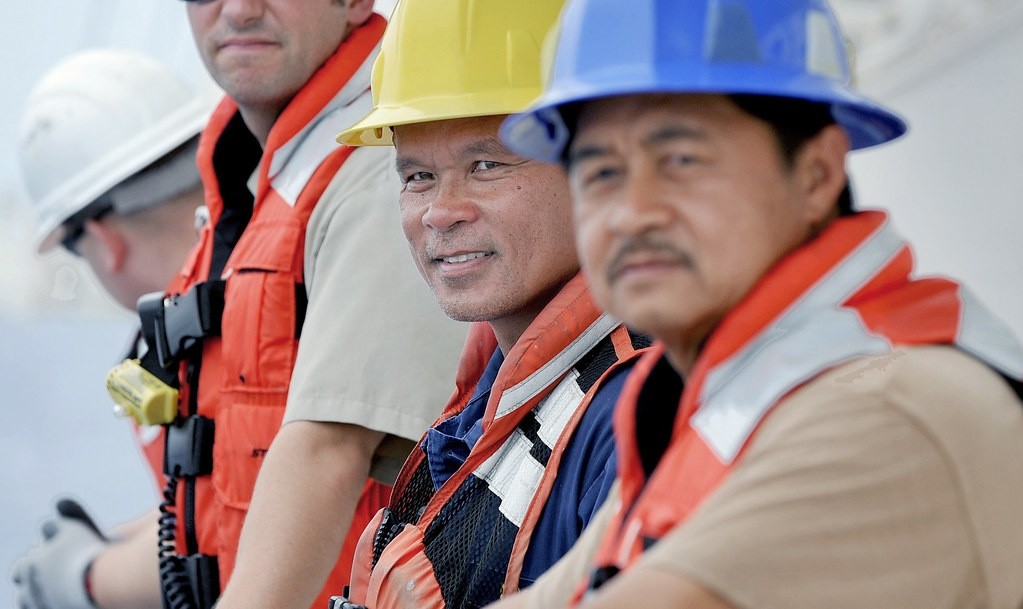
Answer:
[62,205,112,255]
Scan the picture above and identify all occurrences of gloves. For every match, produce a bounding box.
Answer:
[12,499,107,609]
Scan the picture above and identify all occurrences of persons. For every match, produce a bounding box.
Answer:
[13,31,224,609]
[103,0,478,609]
[472,0,1023,609]
[328,0,652,609]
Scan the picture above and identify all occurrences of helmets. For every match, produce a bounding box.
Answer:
[497,0,906,166]
[335,0,567,147]
[18,50,234,258]
